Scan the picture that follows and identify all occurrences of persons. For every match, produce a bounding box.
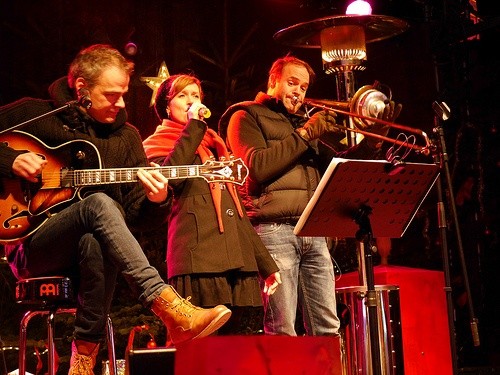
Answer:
[0,44,231,375]
[218,56,402,338]
[141,73,281,334]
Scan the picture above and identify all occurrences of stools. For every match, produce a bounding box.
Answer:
[18,308,118,375]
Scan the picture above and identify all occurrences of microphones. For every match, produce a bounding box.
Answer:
[77,88,92,109]
[199,109,212,118]
